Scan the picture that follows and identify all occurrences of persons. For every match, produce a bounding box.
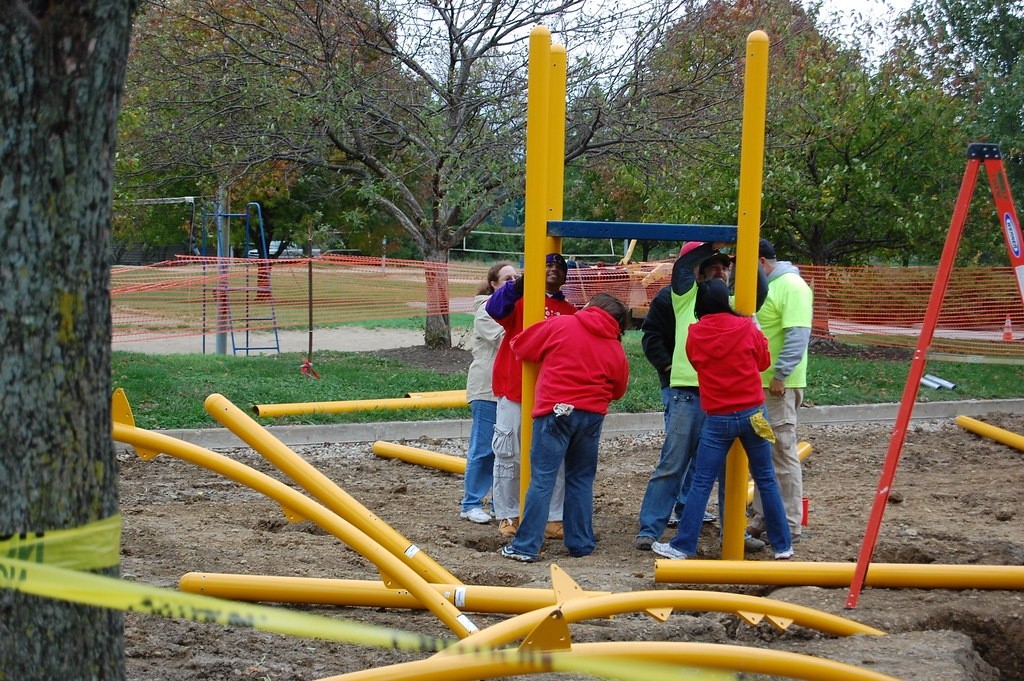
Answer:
[636,242,814,548]
[651,280,793,560]
[501,291,628,562]
[459,253,579,539]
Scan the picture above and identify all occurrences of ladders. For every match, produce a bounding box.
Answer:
[844,143,1024,609]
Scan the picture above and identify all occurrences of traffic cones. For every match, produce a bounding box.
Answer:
[1003,314,1013,341]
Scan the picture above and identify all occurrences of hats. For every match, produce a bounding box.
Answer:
[731,238,776,264]
[700,250,730,271]
[546,253,567,270]
[680,242,703,256]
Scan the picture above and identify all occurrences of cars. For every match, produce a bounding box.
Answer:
[248,241,297,259]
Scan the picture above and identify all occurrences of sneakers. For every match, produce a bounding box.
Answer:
[703,511,716,522]
[545,521,601,541]
[668,516,681,527]
[775,546,794,559]
[502,544,534,562]
[721,536,766,552]
[459,507,492,523]
[635,534,654,550]
[651,541,687,561]
[496,519,517,537]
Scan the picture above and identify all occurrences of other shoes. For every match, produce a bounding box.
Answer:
[759,532,800,542]
[746,525,761,539]
